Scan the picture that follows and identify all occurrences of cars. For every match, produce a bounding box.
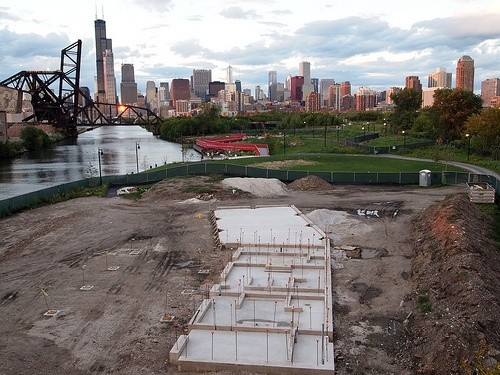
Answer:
[116,186,138,196]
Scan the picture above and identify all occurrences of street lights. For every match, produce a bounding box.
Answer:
[401,128,406,157]
[361,124,366,152]
[383,118,387,137]
[136,141,140,175]
[97,147,104,185]
[466,131,470,161]
[366,121,370,153]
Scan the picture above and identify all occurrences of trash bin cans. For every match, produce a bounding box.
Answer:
[418,170,431,189]
